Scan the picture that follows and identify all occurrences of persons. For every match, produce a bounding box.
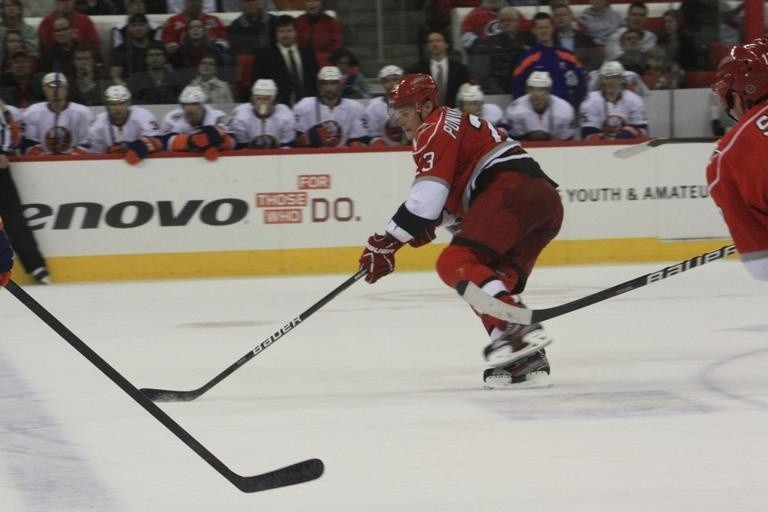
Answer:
[705,36,768,284]
[359,72,563,389]
[0,218,13,288]
[0,100,50,284]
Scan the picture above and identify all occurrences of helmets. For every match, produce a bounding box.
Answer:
[317,66,343,84]
[106,85,132,105]
[386,72,440,114]
[455,82,484,102]
[711,36,768,124]
[377,64,403,80]
[249,77,277,101]
[41,70,70,90]
[176,84,209,106]
[600,59,626,83]
[524,70,554,90]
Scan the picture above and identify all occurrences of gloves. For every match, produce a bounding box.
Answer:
[352,233,402,284]
[106,125,239,168]
[588,125,646,143]
[348,136,388,149]
[298,123,329,148]
[405,224,438,248]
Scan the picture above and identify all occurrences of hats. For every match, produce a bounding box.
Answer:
[128,12,148,27]
[10,48,31,62]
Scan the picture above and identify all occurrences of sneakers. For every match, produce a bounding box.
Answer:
[32,266,49,281]
[478,315,549,359]
[483,348,550,385]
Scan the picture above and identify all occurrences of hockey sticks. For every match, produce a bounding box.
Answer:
[6,283,324,493]
[141,269,364,400]
[455,245,735,327]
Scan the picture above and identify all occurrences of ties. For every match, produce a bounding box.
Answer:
[285,49,302,96]
[434,65,445,107]
